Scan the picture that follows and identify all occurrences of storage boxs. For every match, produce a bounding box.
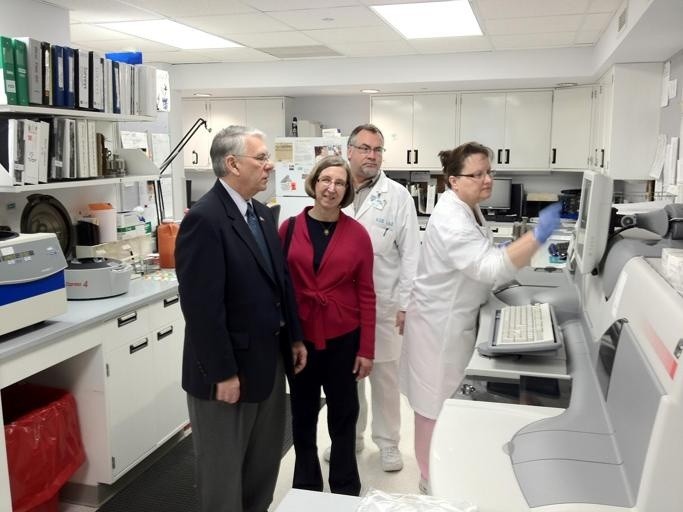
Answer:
[289,118,322,138]
[116,207,150,240]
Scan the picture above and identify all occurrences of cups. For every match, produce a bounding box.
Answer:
[659,248,683,293]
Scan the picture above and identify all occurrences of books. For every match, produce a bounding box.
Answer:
[0,34,157,186]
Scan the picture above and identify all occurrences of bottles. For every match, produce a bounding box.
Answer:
[291,116,298,137]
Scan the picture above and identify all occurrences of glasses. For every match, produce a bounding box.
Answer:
[349,144,385,154]
[234,153,271,164]
[453,170,496,180]
[316,177,348,189]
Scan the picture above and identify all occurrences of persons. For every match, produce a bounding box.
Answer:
[175,125,307,511]
[399,141,562,495]
[277,156,376,497]
[324,124,420,473]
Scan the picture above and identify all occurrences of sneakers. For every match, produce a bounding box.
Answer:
[380,446,403,472]
[324,437,363,461]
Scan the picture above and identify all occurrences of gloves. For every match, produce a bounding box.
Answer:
[535,203,562,243]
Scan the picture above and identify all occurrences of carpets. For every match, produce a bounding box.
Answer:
[89,392,327,512]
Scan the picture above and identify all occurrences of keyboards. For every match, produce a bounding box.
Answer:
[496,303,554,347]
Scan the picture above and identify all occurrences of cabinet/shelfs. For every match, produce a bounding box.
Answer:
[363,89,458,175]
[209,94,290,172]
[0,101,170,196]
[180,97,212,171]
[587,58,664,183]
[457,86,553,178]
[104,289,191,485]
[548,81,593,174]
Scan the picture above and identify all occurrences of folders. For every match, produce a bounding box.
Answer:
[112,62,120,114]
[51,45,75,107]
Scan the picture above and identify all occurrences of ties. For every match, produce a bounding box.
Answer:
[246,202,277,280]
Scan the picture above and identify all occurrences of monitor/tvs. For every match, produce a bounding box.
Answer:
[479,178,512,220]
[574,170,614,274]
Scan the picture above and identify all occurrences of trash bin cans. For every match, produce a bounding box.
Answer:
[0,383,75,512]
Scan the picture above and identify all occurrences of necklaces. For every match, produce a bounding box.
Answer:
[311,208,340,236]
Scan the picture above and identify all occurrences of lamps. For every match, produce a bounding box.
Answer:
[154,116,221,229]
